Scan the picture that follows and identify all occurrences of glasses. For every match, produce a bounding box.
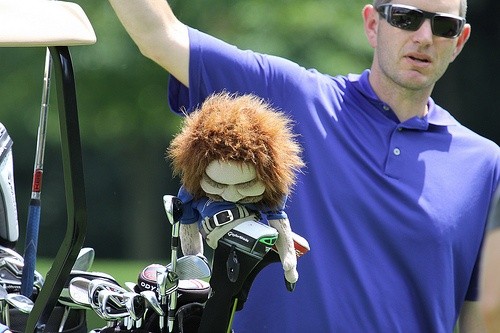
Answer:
[375,3,465,38]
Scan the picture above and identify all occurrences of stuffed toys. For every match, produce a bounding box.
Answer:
[166,89,307,293]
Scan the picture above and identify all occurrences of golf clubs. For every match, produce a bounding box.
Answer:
[22,47,52,297]
[0,194,215,333]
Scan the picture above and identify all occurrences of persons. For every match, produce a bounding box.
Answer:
[106,0,500,333]
